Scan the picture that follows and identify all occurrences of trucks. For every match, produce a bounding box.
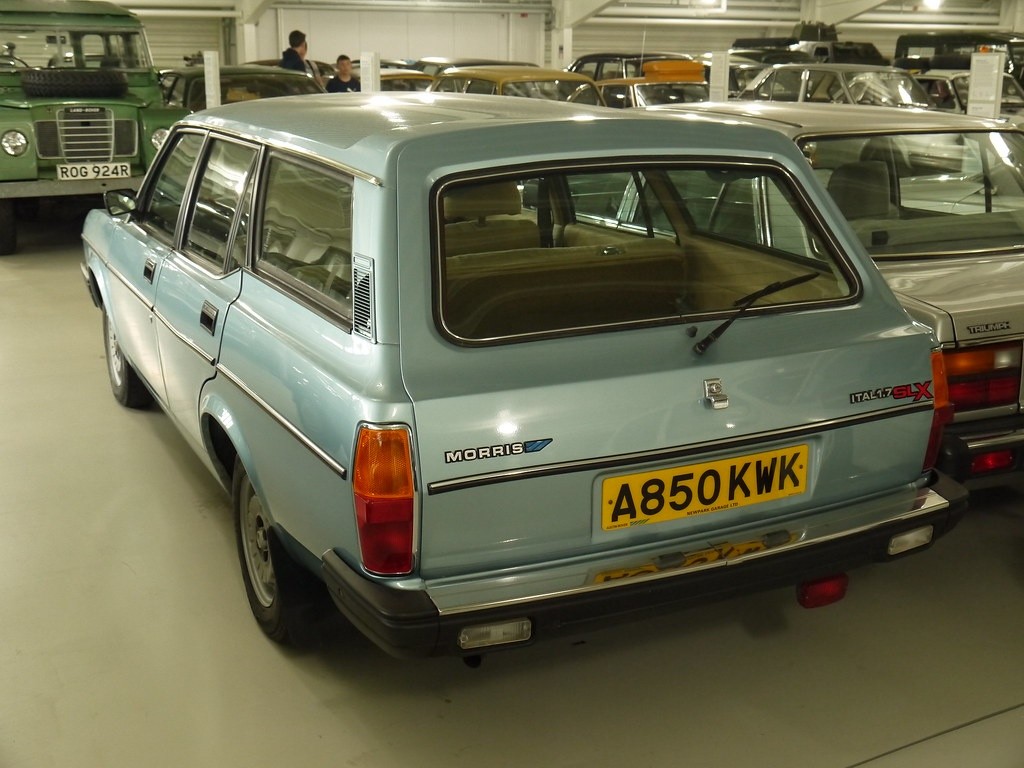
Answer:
[0,0,195,258]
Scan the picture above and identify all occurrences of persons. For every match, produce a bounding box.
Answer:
[279,29,328,93]
[325,55,361,93]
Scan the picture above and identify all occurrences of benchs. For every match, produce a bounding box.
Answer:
[443,241,688,339]
[847,210,1024,250]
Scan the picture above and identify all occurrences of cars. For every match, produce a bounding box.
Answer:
[517,98,1023,504]
[79,93,968,660]
[151,38,1024,213]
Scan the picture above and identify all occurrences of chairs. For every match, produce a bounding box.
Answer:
[227,86,261,101]
[100,57,121,67]
[445,183,540,254]
[826,160,901,220]
